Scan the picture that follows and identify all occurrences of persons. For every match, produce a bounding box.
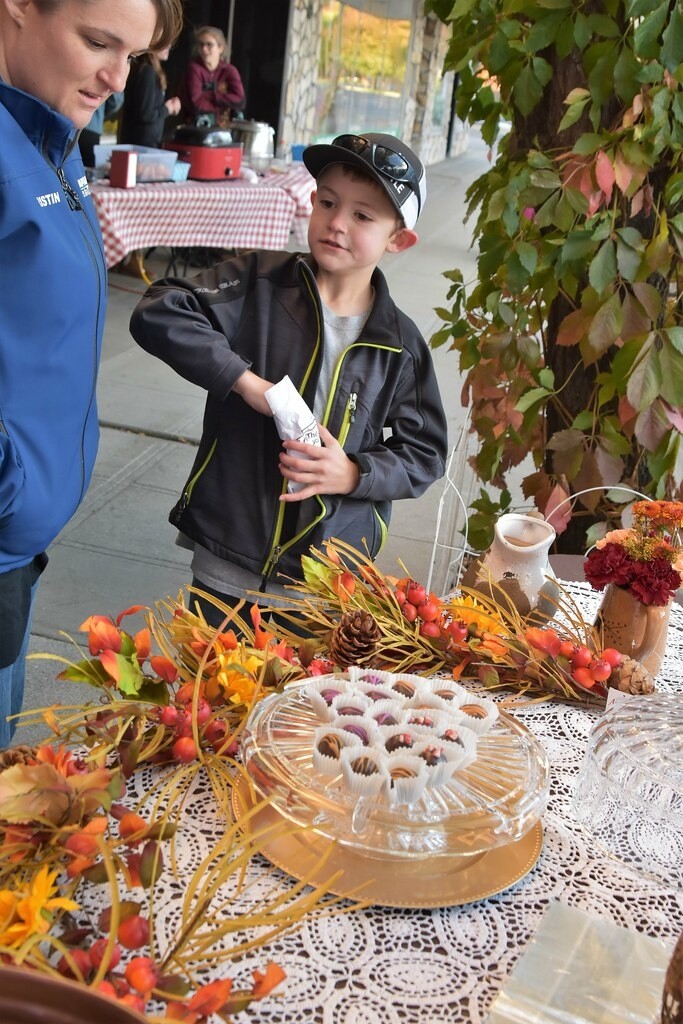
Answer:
[0,0,181,763]
[77,26,246,180]
[130,135,449,639]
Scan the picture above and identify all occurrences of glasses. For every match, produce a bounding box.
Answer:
[331,134,422,221]
[198,40,218,48]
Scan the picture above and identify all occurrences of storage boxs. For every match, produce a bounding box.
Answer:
[93,144,178,182]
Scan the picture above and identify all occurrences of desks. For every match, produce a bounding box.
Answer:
[0,577,683,1024]
[91,181,297,286]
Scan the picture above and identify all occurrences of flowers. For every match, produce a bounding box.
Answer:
[583,500,683,607]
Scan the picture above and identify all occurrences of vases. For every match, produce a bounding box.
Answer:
[461,513,559,634]
[593,583,670,680]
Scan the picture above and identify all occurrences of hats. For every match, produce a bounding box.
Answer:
[303,132,426,231]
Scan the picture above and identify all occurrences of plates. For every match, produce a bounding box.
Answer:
[230,763,542,910]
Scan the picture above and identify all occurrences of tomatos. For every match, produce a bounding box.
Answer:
[55,915,198,1024]
[160,702,239,760]
[390,587,467,642]
[559,642,621,688]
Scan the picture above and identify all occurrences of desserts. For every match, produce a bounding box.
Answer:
[316,675,489,796]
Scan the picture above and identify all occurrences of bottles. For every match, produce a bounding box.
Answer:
[461,513,561,629]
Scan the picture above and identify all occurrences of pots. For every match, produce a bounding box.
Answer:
[159,116,243,182]
[216,116,275,172]
